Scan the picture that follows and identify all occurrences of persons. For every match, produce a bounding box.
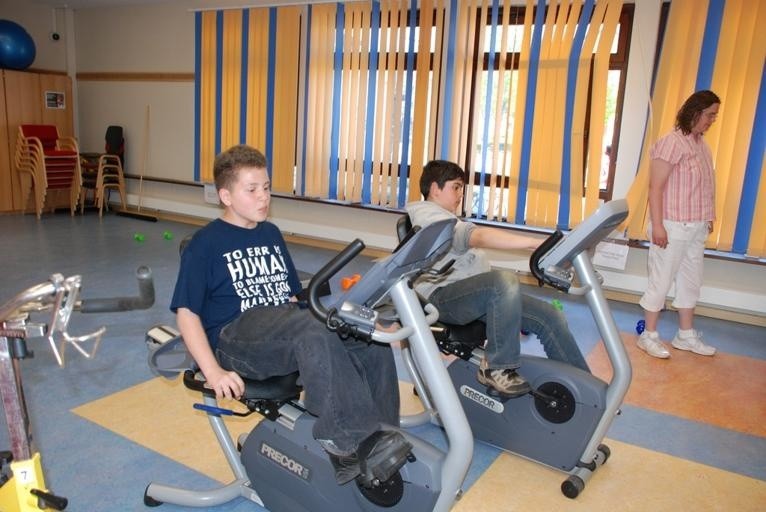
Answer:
[636,89,722,359]
[406,159,593,397]
[169,143,407,487]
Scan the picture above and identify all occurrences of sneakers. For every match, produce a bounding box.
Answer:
[331,430,406,485]
[672,334,716,355]
[477,358,532,395]
[638,332,669,358]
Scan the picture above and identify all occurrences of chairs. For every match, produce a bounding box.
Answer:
[13,123,126,221]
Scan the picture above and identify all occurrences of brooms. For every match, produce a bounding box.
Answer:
[115,102,158,223]
